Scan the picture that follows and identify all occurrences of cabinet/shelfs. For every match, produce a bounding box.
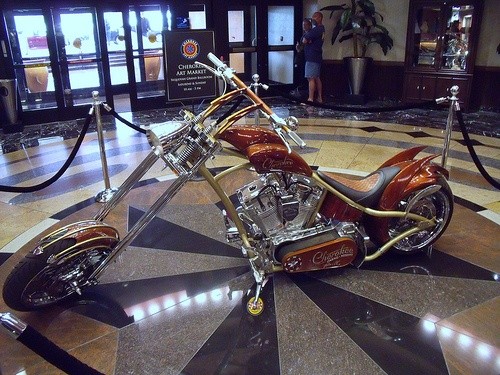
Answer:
[402,0,484,111]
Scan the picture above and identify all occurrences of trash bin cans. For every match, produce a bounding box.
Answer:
[0,78,19,133]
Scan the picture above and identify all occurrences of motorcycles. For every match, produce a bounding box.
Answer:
[2,52,456,313]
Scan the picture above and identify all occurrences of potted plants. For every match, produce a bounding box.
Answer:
[320,0,393,95]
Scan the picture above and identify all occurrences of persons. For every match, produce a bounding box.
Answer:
[296,18,312,106]
[303,12,325,103]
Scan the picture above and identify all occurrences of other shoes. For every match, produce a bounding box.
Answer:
[300,101,314,105]
[314,101,323,106]
